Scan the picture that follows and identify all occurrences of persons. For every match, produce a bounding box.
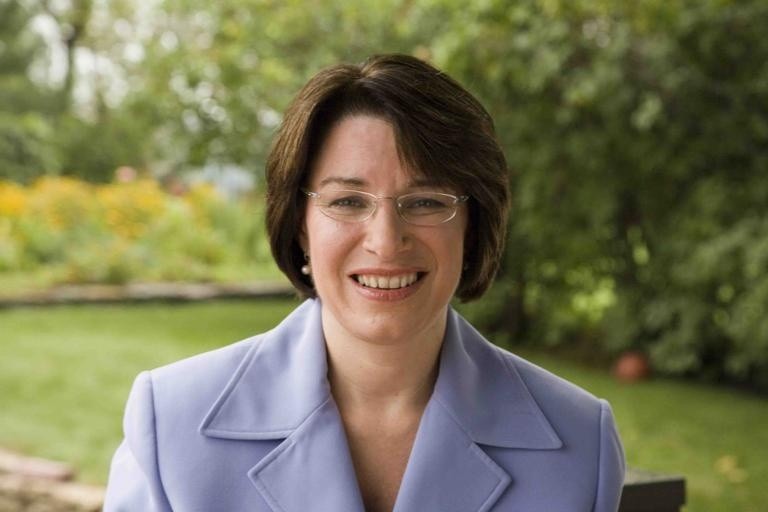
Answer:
[102,52,624,512]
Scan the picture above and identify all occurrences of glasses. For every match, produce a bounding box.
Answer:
[301,185,470,227]
